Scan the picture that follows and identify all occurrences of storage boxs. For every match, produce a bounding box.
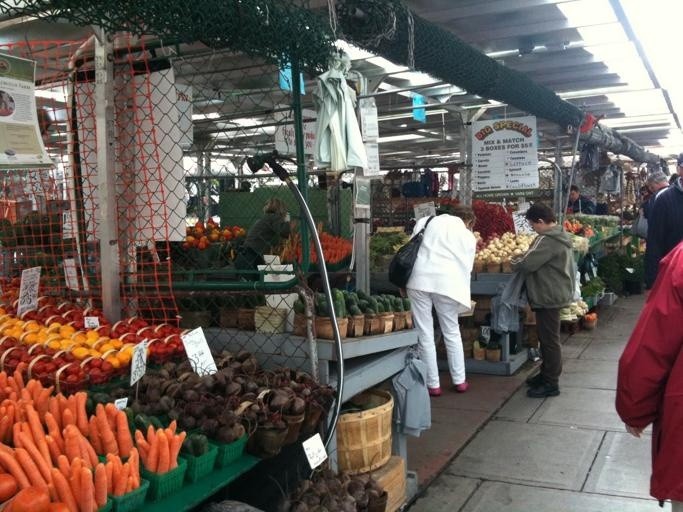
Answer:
[349,455,407,512]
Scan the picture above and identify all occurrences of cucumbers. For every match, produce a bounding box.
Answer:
[294,287,410,316]
[86,391,207,457]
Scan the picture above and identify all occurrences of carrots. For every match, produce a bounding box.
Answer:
[283,230,352,263]
[0,362,187,512]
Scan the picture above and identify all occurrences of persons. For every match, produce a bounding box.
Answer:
[388,204,478,395]
[567,185,596,214]
[634,153,682,304]
[243,197,292,281]
[616,238,682,512]
[507,200,578,399]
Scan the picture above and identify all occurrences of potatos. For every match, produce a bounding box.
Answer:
[476,231,537,264]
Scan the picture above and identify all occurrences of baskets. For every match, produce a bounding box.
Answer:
[88,426,247,512]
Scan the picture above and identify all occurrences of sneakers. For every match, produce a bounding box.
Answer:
[527,382,560,397]
[525,373,543,385]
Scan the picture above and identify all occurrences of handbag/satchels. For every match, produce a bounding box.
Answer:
[633,216,648,240]
[389,230,423,287]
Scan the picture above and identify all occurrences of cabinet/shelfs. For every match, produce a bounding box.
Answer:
[193,326,419,511]
[434,231,623,376]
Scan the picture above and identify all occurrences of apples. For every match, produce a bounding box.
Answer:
[0,277,184,393]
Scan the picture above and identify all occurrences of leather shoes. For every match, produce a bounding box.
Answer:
[428,387,440,396]
[455,382,469,392]
